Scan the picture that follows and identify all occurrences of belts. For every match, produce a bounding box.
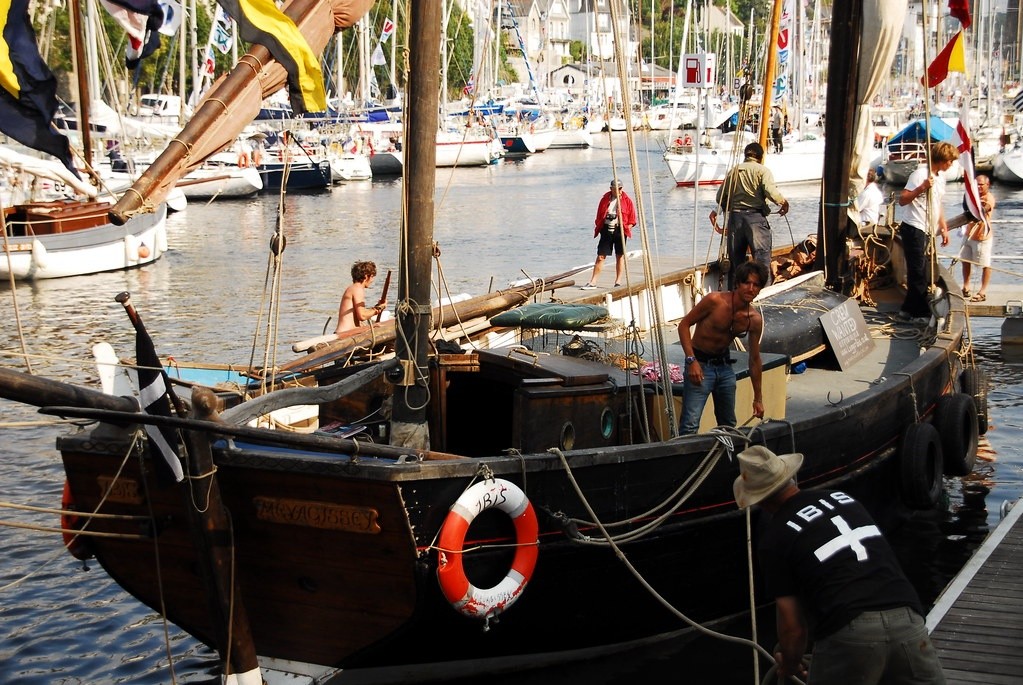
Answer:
[697,357,737,363]
[731,209,758,213]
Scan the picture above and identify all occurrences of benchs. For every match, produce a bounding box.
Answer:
[489,303,626,361]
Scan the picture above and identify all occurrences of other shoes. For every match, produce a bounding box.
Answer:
[899,311,929,323]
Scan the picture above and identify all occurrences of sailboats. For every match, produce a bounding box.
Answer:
[0,0,1023,685]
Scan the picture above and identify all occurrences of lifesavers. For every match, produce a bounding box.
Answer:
[303,145,313,156]
[437,477,539,622]
[906,424,944,508]
[238,152,249,168]
[966,368,988,435]
[942,394,979,476]
[253,150,261,167]
[279,150,291,162]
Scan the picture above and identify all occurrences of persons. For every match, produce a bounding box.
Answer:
[858,169,884,227]
[733,444,947,685]
[957,175,995,301]
[333,260,388,365]
[896,141,959,323]
[581,179,637,290]
[678,261,768,436]
[772,104,784,154]
[715,142,789,291]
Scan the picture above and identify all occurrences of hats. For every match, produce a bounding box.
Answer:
[773,105,781,109]
[733,445,803,508]
[610,179,623,188]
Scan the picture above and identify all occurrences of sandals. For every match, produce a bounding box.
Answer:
[961,289,971,297]
[970,293,986,302]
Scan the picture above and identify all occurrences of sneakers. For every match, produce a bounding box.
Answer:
[614,283,620,288]
[581,283,597,290]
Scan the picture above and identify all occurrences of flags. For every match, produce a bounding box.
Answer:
[949,101,990,240]
[136,312,185,484]
[100,0,164,70]
[215,0,327,113]
[370,17,394,67]
[773,14,789,100]
[920,0,973,89]
[0,0,84,183]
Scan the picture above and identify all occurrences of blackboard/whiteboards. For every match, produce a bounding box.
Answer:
[819,297,876,372]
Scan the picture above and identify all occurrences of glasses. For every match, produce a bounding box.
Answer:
[729,319,751,338]
